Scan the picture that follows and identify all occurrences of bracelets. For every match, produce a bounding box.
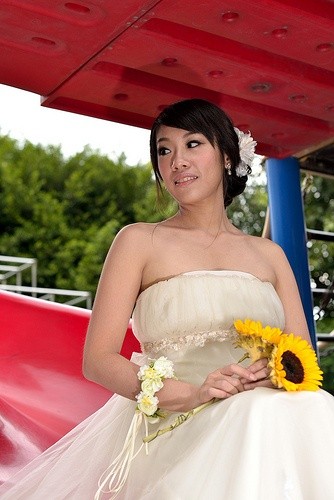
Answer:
[136,354,177,416]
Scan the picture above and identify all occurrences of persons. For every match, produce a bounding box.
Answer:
[0,99,334,499]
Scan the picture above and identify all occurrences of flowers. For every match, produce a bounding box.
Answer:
[133,355,174,419]
[142,318,324,445]
[233,127,257,178]
